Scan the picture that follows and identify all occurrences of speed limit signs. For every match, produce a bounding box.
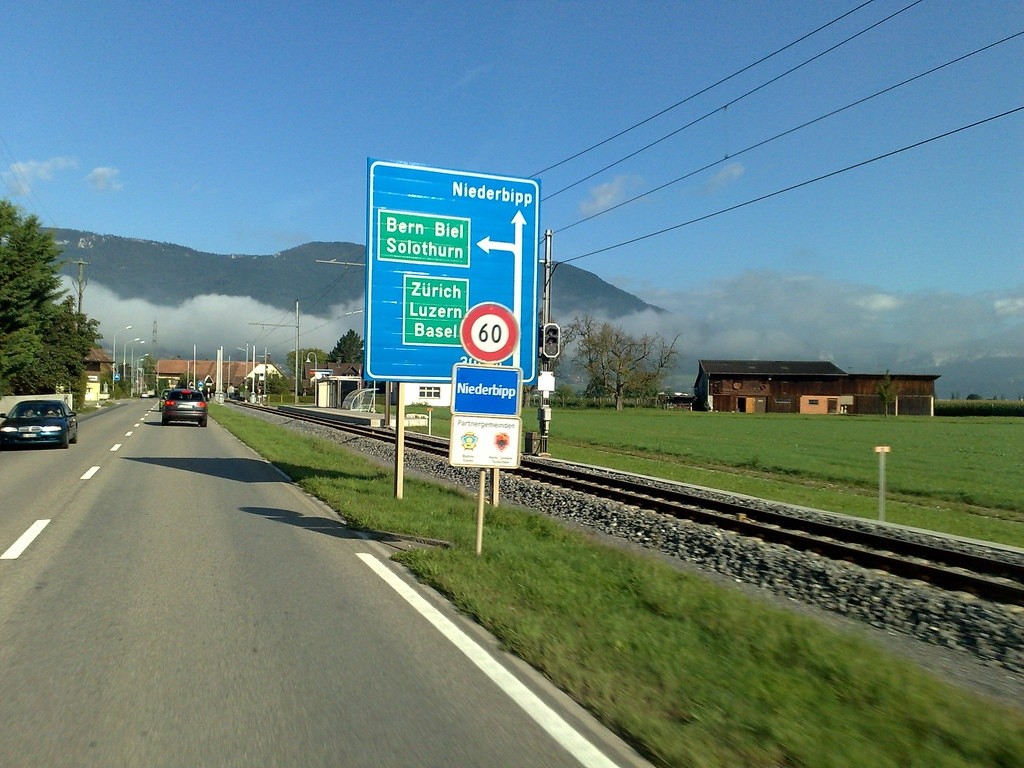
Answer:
[460,303,520,364]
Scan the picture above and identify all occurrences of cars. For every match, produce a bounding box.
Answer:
[161,388,208,427]
[0,400,77,449]
[159,389,172,412]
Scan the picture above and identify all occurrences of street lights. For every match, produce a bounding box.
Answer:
[112,325,132,391]
[236,343,248,404]
[123,338,141,378]
[136,353,149,394]
[131,341,146,385]
[247,299,301,406]
[133,358,144,369]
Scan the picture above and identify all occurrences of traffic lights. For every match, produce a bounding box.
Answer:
[543,322,561,359]
